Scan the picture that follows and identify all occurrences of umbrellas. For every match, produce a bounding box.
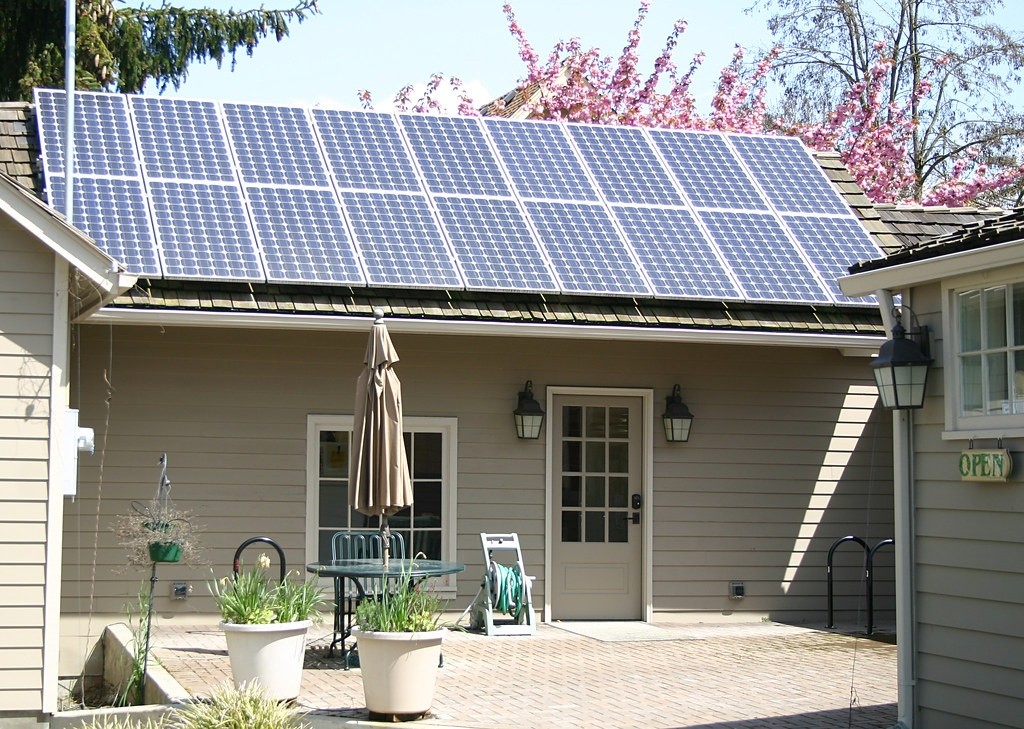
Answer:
[347,310,415,588]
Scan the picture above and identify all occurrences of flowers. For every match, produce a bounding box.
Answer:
[205,553,338,630]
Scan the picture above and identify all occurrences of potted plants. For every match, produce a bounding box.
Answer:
[106,495,210,576]
[351,552,470,722]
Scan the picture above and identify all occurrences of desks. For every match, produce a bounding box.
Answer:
[306,558,464,671]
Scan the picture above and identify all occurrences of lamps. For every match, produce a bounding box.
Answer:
[512,380,546,440]
[869,304,935,410]
[661,383,694,443]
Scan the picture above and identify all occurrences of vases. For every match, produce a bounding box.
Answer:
[219,620,311,701]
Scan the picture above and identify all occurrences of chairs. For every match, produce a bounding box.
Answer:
[332,531,404,651]
[470,532,536,636]
